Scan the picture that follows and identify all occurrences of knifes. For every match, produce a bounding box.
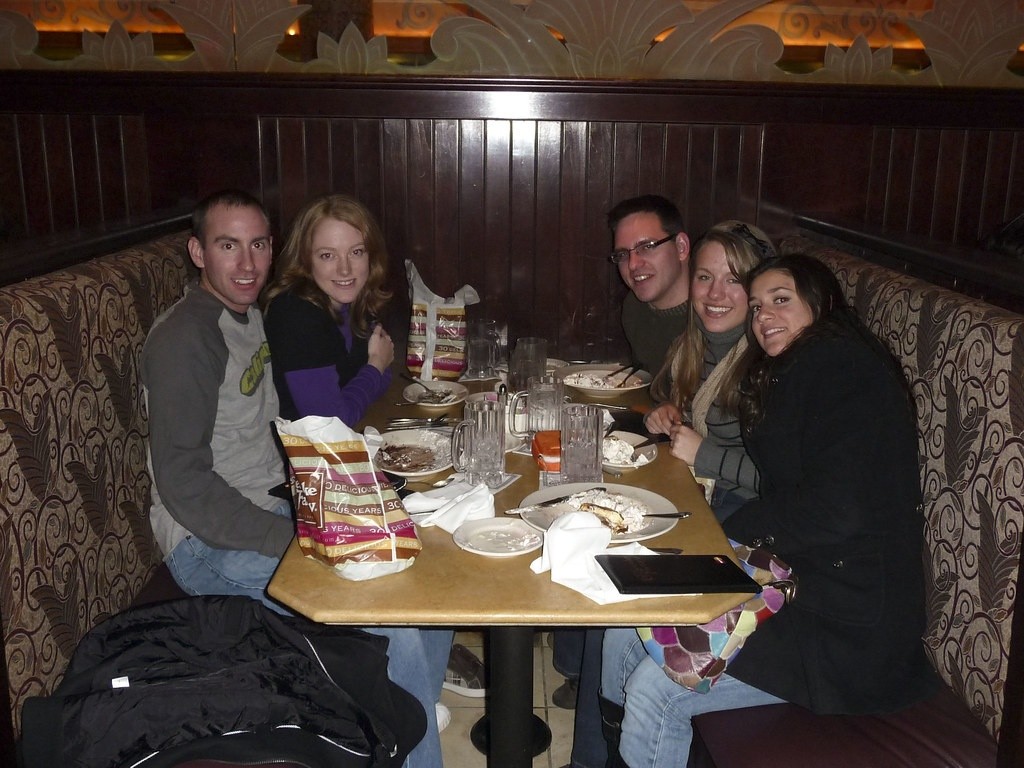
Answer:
[504,488,607,515]
[385,415,457,431]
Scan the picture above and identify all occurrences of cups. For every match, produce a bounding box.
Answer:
[509,377,564,450]
[510,337,548,401]
[467,318,502,380]
[560,404,604,484]
[451,402,505,488]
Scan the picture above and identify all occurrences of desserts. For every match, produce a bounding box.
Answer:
[597,434,650,467]
[568,489,647,534]
[563,374,624,390]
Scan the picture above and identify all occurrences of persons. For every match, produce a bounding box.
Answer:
[609,195,697,392]
[549,218,782,768]
[138,189,455,768]
[597,251,924,768]
[254,193,492,699]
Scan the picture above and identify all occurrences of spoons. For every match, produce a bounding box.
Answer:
[391,413,448,424]
[614,363,643,387]
[400,372,445,400]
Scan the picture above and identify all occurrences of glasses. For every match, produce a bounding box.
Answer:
[611,235,677,263]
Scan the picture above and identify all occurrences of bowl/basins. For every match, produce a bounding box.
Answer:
[601,431,658,475]
[551,364,653,399]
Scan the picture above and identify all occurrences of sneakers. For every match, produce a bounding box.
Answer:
[435,702,451,734]
[443,644,486,698]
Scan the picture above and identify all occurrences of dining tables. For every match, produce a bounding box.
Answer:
[264,354,756,768]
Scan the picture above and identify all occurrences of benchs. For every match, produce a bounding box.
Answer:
[687,206,1023,768]
[0,201,308,768]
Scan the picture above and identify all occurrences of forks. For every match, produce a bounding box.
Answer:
[393,399,445,406]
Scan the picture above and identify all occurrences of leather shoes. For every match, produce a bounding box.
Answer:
[553,675,580,709]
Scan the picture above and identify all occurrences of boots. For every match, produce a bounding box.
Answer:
[597,686,629,768]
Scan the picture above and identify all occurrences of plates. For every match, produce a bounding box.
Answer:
[403,381,469,407]
[374,429,456,476]
[464,391,522,406]
[519,482,679,544]
[544,358,569,377]
[452,518,545,558]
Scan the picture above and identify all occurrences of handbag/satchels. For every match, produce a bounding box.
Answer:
[275,415,422,581]
[637,537,794,694]
[405,258,480,384]
